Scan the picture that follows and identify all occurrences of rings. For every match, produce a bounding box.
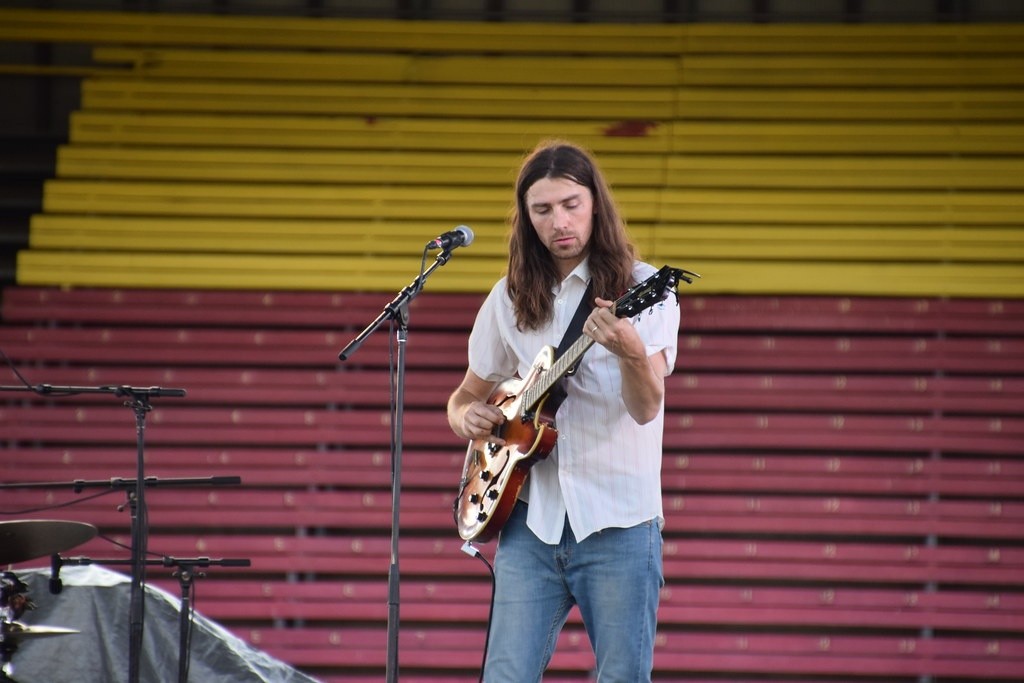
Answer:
[592,326,599,334]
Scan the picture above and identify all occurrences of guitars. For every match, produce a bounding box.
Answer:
[452,263,700,543]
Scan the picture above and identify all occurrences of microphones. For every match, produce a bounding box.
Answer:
[427,225,474,253]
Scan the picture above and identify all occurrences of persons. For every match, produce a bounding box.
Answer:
[445,140,682,683]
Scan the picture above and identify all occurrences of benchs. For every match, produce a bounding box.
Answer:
[1,1,1024,683]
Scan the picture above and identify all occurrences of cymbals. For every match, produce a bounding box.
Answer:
[0,519,98,565]
[9,625,80,638]
